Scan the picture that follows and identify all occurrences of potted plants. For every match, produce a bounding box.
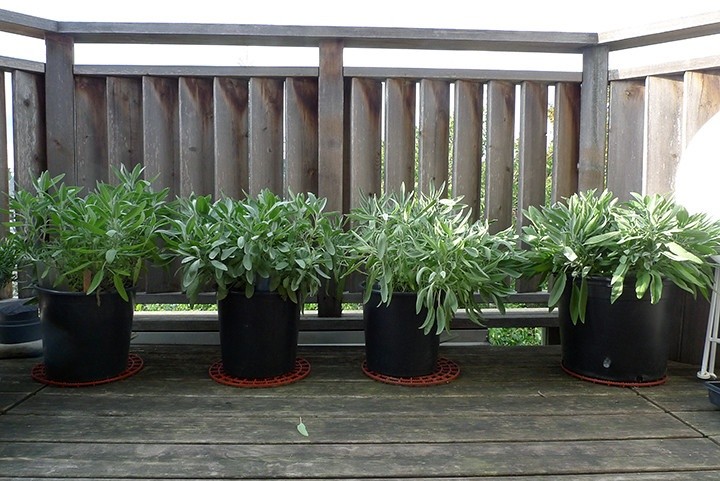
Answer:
[0,160,182,383]
[331,181,528,379]
[158,187,342,381]
[512,184,720,386]
